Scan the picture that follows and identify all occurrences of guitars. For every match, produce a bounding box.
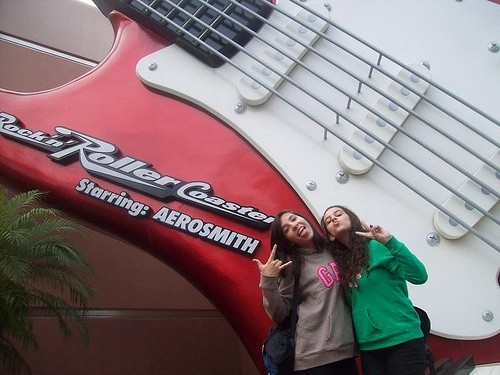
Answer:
[0,0,500,373]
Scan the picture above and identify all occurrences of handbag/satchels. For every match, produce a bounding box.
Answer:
[262,318,295,375]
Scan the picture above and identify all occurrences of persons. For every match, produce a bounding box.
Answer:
[244,210,359,375]
[318,204,434,375]
